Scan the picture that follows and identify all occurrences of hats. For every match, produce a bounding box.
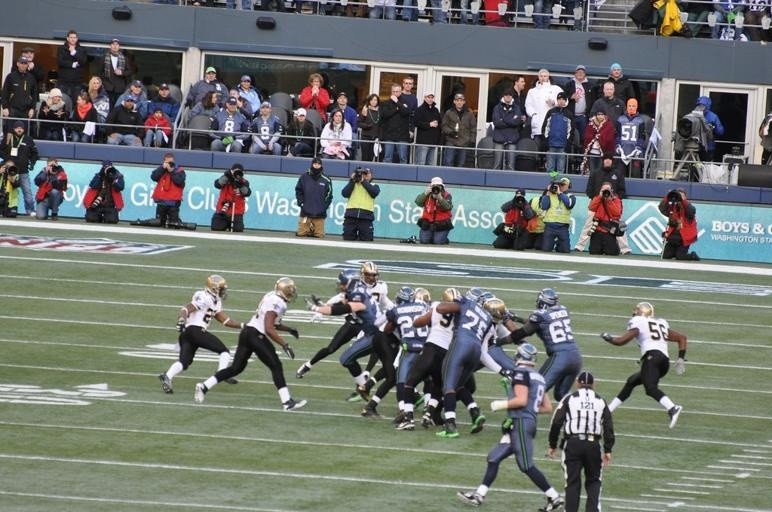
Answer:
[423,91,435,97]
[17,56,28,64]
[159,83,169,90]
[260,101,271,108]
[227,96,237,105]
[153,107,164,114]
[594,107,607,117]
[337,92,349,98]
[576,372,594,386]
[611,63,621,71]
[241,75,251,82]
[110,38,120,44]
[124,95,135,101]
[502,88,515,96]
[21,46,35,52]
[296,108,306,118]
[455,93,465,101]
[575,65,586,71]
[206,66,217,74]
[557,92,567,101]
[131,80,144,87]
[426,177,573,197]
[49,88,62,98]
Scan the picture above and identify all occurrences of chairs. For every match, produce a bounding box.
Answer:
[28,91,653,182]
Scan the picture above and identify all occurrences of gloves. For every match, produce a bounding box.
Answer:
[176,318,184,333]
[490,399,508,413]
[303,293,325,311]
[673,357,686,375]
[488,334,502,348]
[601,332,613,342]
[287,326,299,339]
[283,343,295,360]
[366,123,372,129]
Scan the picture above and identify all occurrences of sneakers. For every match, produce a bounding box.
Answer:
[296,360,314,380]
[222,375,238,385]
[193,382,208,405]
[282,397,308,411]
[334,378,486,438]
[666,404,683,429]
[456,491,485,507]
[690,251,701,262]
[158,372,174,395]
[538,494,566,512]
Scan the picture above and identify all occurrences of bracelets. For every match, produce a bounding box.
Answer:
[677,350,687,361]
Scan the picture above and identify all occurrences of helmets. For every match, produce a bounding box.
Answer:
[206,274,227,295]
[635,302,654,318]
[274,277,296,300]
[516,343,537,362]
[338,260,560,318]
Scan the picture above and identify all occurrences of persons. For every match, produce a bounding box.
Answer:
[191,275,308,413]
[157,273,249,395]
[543,370,616,512]
[456,342,566,512]
[600,301,689,430]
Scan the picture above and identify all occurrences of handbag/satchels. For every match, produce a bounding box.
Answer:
[609,219,628,237]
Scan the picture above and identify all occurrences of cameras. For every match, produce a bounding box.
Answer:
[169,162,175,167]
[432,186,440,194]
[52,165,62,172]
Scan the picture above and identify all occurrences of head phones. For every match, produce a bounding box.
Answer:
[569,183,573,190]
